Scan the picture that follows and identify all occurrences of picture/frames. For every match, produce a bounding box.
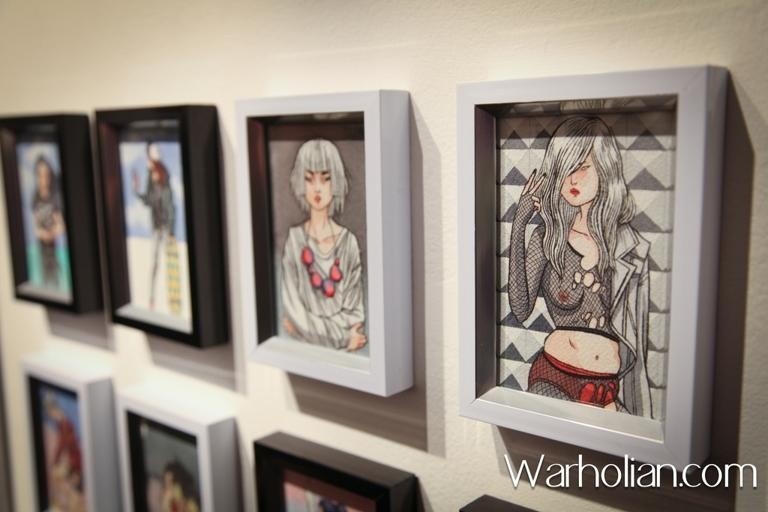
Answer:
[251,431,417,512]
[239,88,418,400]
[1,111,105,315]
[114,382,241,511]
[19,355,118,511]
[460,61,728,471]
[94,105,230,350]
